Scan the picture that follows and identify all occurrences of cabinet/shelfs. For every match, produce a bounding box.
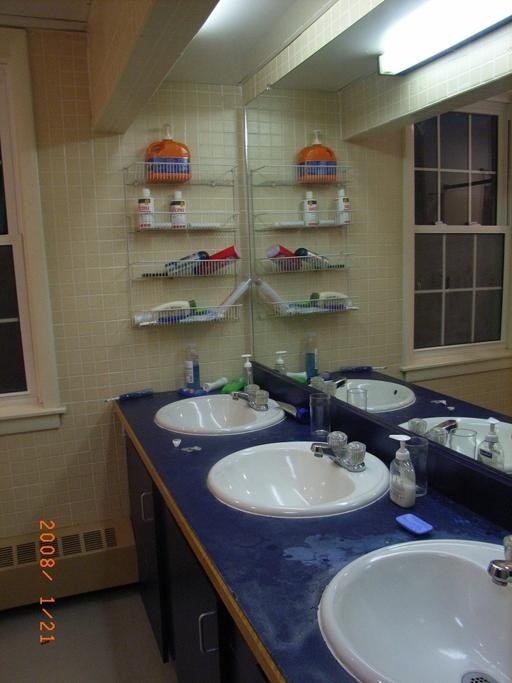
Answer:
[120,160,246,328]
[248,162,361,322]
[112,396,240,683]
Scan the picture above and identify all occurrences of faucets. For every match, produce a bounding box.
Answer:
[486,534,512,587]
[423,419,458,448]
[231,383,270,412]
[312,375,347,398]
[310,430,367,472]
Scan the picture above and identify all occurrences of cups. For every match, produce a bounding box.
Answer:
[347,388,367,412]
[406,437,429,497]
[311,394,331,437]
[449,428,477,460]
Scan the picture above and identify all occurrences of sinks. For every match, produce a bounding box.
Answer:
[206,441,390,520]
[335,379,417,414]
[315,539,512,683]
[153,393,288,437]
[396,416,512,476]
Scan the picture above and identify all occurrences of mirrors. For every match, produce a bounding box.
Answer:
[236,1,512,476]
